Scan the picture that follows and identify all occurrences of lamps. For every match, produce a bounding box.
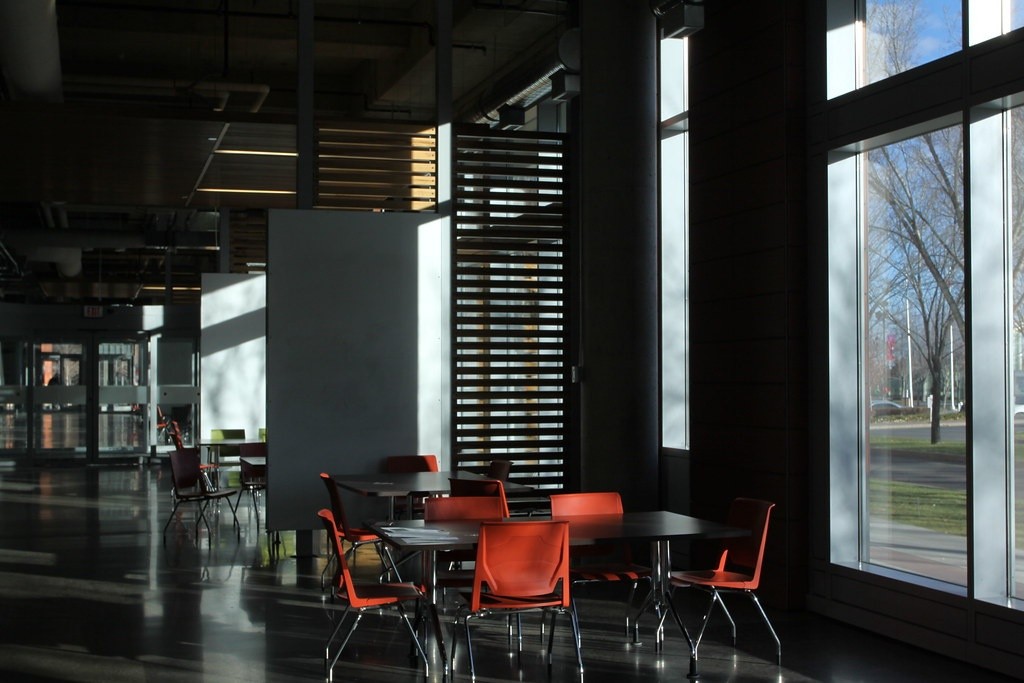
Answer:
[493,0,706,130]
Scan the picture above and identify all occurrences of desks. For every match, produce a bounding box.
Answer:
[329,471,536,583]
[196,439,267,504]
[362,511,753,678]
[239,456,266,470]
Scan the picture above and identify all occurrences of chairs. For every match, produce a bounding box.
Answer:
[234,471,266,534]
[541,493,655,647]
[157,406,172,443]
[318,507,430,683]
[170,421,266,507]
[655,496,784,671]
[320,455,524,654]
[164,448,240,550]
[451,521,583,683]
